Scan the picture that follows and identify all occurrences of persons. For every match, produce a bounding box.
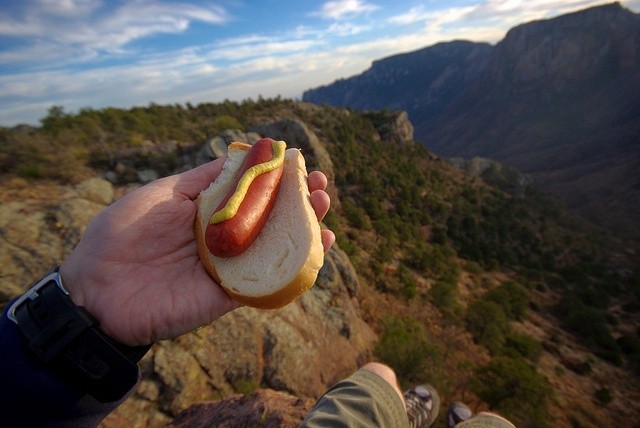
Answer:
[1,147,516,428]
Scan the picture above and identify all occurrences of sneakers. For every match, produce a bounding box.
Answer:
[447,401,472,428]
[403,384,440,428]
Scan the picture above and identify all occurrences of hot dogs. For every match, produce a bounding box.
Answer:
[193,136,322,310]
